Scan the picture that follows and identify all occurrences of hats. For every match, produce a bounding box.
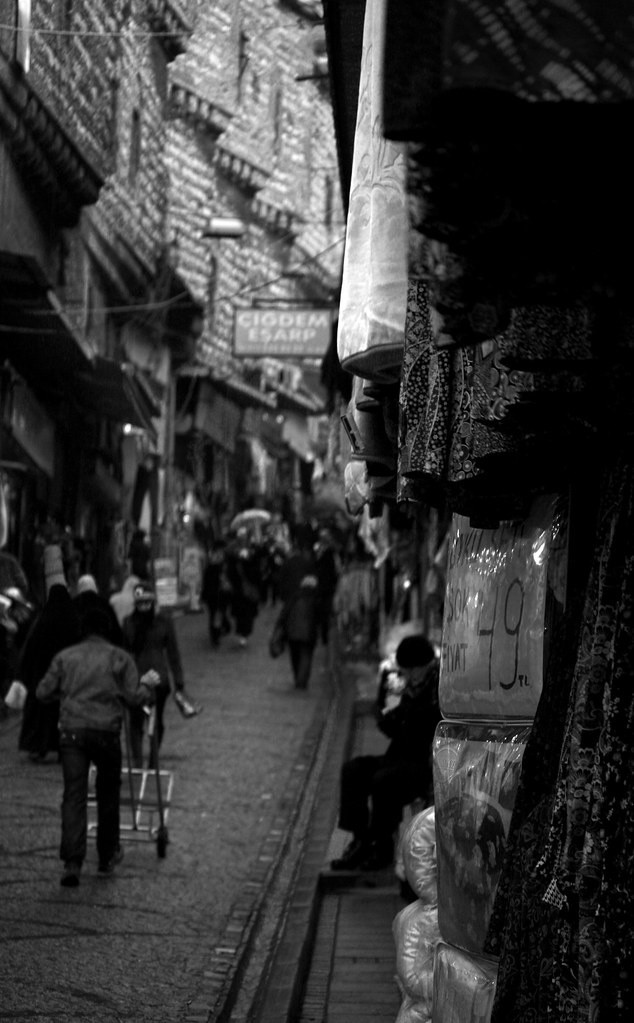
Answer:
[132,582,156,604]
[396,635,434,670]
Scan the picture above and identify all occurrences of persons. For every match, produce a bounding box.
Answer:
[329,632,440,871]
[36,608,155,884]
[19,509,382,770]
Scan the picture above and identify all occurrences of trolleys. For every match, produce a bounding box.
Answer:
[87,692,178,860]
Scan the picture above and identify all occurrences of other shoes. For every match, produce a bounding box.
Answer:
[59,861,82,888]
[98,846,124,874]
[331,831,394,872]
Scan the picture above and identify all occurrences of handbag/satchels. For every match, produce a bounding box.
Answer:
[4,682,28,709]
[270,624,286,657]
